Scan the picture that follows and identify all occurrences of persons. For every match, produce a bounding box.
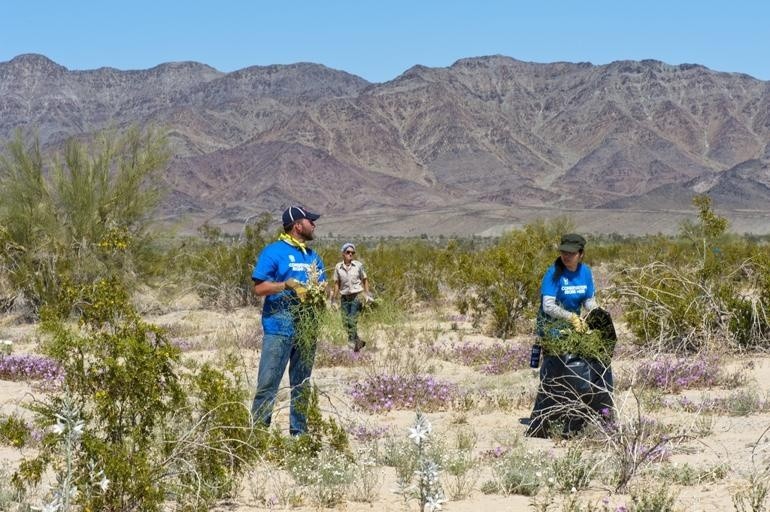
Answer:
[251,204,328,438]
[531,235,595,419]
[331,243,371,352]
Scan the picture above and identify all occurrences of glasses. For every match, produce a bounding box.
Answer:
[345,249,355,254]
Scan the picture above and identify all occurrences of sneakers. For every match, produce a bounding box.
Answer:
[353,340,366,353]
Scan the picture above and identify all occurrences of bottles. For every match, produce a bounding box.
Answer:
[530,338,541,369]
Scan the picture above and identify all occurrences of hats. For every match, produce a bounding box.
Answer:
[341,242,355,253]
[281,204,321,228]
[559,233,586,253]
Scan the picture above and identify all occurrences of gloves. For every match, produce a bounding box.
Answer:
[570,313,589,335]
